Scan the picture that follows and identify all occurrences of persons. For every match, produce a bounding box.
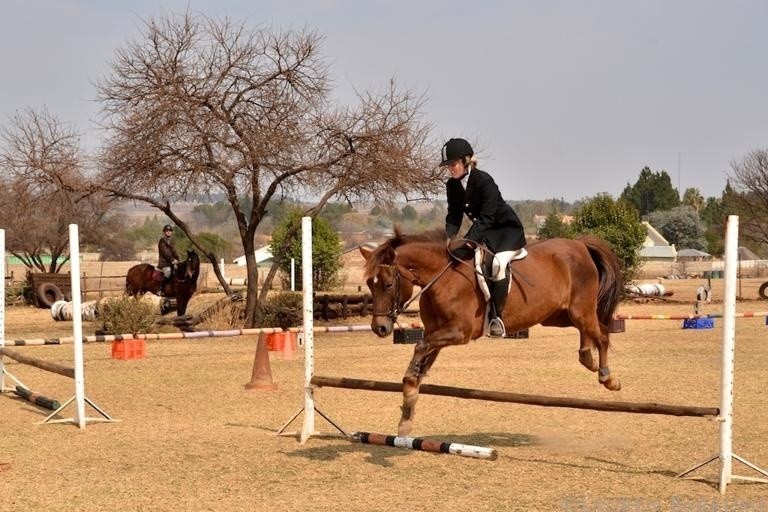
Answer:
[440,137,527,337]
[157,224,178,296]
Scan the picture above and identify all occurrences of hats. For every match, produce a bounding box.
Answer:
[439,138,473,166]
[163,224,172,232]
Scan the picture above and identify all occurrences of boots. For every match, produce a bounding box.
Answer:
[157,276,169,296]
[489,276,508,336]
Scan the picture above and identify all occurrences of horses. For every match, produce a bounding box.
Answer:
[123,248,202,317]
[358,225,624,438]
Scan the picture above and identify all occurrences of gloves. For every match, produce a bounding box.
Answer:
[450,244,473,266]
